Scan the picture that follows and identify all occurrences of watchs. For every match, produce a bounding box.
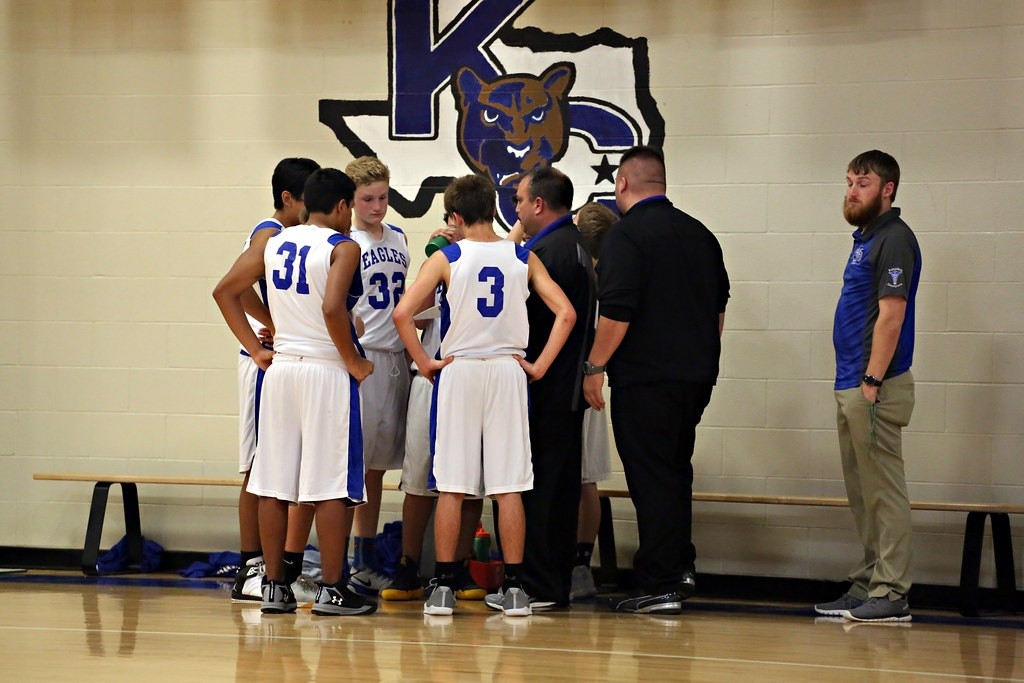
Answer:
[862,374,882,387]
[583,362,608,375]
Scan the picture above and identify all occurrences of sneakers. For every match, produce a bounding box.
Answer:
[456,601,491,617]
[259,611,297,629]
[483,584,562,611]
[241,609,311,625]
[501,616,529,642]
[675,570,695,600]
[230,565,314,608]
[310,584,378,616]
[261,576,298,614]
[311,612,379,639]
[423,616,453,638]
[569,567,598,599]
[422,583,455,616]
[814,619,850,632]
[844,590,912,623]
[381,553,424,598]
[382,597,425,619]
[814,594,863,615]
[503,587,531,617]
[843,615,910,646]
[453,566,486,598]
[608,590,682,616]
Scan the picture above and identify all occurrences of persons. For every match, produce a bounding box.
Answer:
[584,145,730,614]
[814,149,923,621]
[211,157,619,615]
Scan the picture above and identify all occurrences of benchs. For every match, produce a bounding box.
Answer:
[595,487,1024,628]
[33,472,403,579]
